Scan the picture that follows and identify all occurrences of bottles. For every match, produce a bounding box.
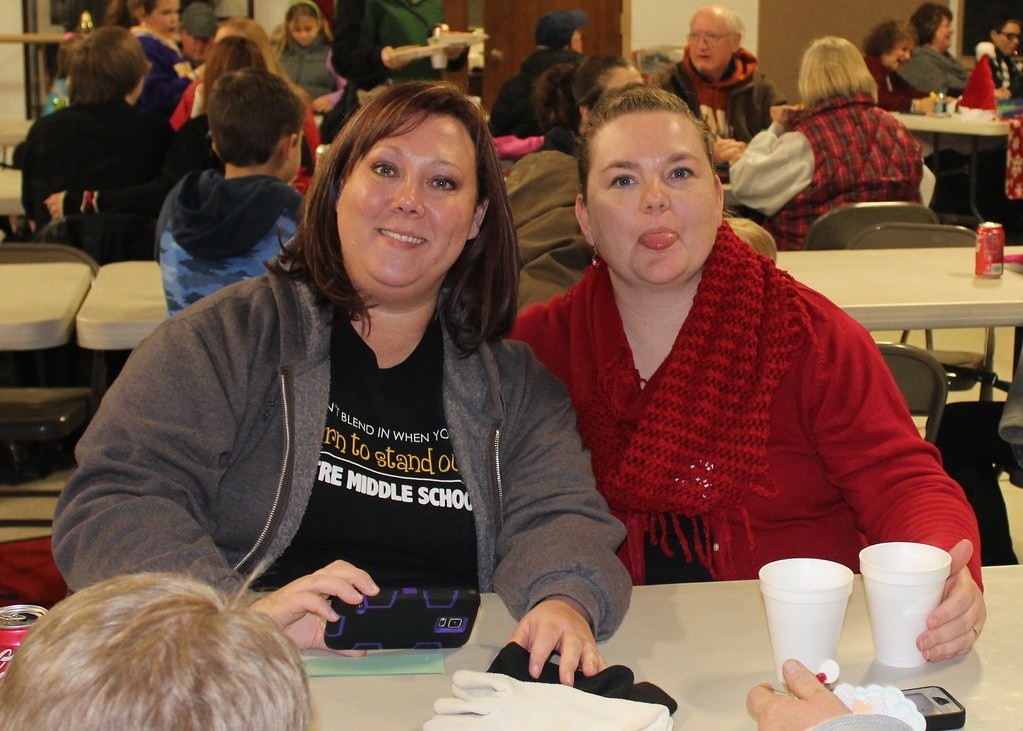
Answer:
[933,88,946,113]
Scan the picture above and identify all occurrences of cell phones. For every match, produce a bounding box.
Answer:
[324,588,481,649]
[903,685,966,731]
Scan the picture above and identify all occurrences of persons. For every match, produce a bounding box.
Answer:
[0,572,313,731]
[501,83,987,661]
[13,0,1022,314]
[746,658,913,731]
[52,80,631,687]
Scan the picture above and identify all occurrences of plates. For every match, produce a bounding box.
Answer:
[432,33,489,46]
[390,44,451,62]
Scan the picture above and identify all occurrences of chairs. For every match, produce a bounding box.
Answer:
[808,204,993,445]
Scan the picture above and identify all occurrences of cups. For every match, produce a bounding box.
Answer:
[427,37,449,68]
[859,542,951,668]
[758,558,854,683]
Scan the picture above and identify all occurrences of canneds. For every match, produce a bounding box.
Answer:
[974,221,1005,279]
[0,604,50,684]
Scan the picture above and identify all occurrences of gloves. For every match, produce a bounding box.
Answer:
[421,671,674,731]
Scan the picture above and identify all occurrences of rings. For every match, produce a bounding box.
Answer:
[971,628,978,641]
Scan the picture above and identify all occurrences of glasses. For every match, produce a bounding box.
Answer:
[996,30,1021,41]
[686,31,731,44]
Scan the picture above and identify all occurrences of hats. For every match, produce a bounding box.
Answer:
[534,9,587,47]
[180,3,216,36]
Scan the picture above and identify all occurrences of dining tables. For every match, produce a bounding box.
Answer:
[0,242,1023,400]
[895,109,1006,225]
[305,564,1023,731]
